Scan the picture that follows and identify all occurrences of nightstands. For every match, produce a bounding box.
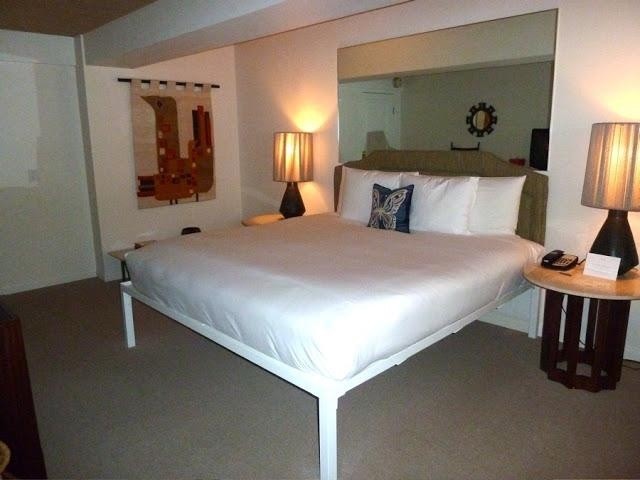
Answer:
[241,213,284,226]
[522,261,640,393]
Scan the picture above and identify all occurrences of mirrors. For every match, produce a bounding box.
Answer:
[466,103,497,136]
[337,8,558,171]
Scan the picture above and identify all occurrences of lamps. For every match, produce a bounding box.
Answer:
[274,132,313,219]
[581,123,640,278]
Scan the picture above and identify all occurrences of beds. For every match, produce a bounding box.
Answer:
[120,150,549,480]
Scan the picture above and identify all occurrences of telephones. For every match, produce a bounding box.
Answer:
[541,250,578,271]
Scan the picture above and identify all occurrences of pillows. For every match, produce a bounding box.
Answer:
[337,166,527,236]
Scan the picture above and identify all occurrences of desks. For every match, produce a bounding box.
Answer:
[108,248,136,282]
